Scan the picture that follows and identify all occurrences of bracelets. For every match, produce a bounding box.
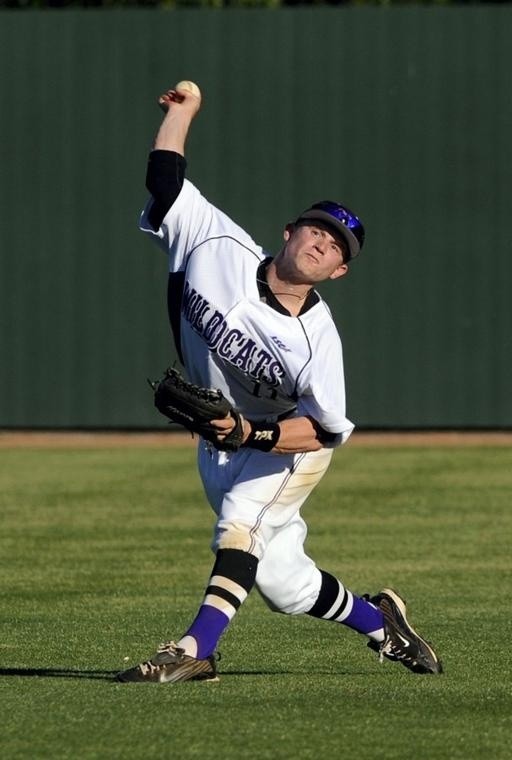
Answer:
[241,418,281,453]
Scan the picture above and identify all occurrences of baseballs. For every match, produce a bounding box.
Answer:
[176,80,200,98]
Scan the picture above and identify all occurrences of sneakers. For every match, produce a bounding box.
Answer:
[363,589,442,674]
[117,640,219,683]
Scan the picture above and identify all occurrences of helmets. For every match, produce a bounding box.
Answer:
[298,201,365,258]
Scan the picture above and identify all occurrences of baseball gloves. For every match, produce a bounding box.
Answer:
[153,368,245,452]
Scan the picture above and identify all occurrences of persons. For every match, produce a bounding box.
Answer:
[112,87,446,686]
